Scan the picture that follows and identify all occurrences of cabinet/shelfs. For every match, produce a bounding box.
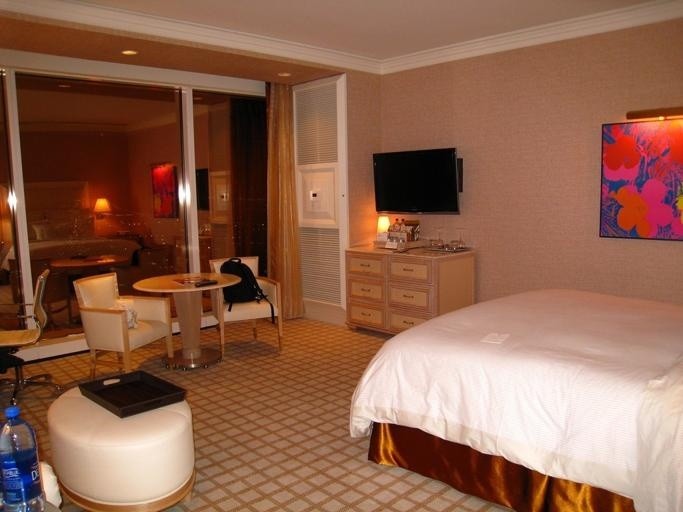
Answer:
[345,241,477,338]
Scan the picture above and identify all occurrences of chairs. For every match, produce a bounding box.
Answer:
[72,255,284,380]
[0,269,64,408]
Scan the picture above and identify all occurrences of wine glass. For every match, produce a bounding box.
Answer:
[455,227,467,250]
[433,226,446,249]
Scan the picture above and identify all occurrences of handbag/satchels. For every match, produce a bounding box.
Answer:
[221,258,262,303]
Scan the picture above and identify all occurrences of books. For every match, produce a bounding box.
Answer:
[173,276,206,286]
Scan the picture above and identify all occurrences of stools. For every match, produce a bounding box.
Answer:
[46,385,197,512]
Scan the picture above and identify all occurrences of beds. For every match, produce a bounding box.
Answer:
[349,287,683,512]
[3,211,137,278]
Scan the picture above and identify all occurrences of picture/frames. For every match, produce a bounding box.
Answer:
[598,119,683,243]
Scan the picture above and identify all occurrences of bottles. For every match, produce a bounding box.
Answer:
[393,218,406,233]
[0,406,47,511]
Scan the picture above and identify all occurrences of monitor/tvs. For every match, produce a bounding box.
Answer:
[372,146,463,215]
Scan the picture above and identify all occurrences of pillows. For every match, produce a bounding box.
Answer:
[32,223,53,240]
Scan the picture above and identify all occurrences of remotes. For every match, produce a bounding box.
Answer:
[69,253,88,259]
[194,279,217,287]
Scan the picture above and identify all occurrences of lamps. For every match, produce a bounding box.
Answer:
[94,198,111,213]
[376,216,390,241]
